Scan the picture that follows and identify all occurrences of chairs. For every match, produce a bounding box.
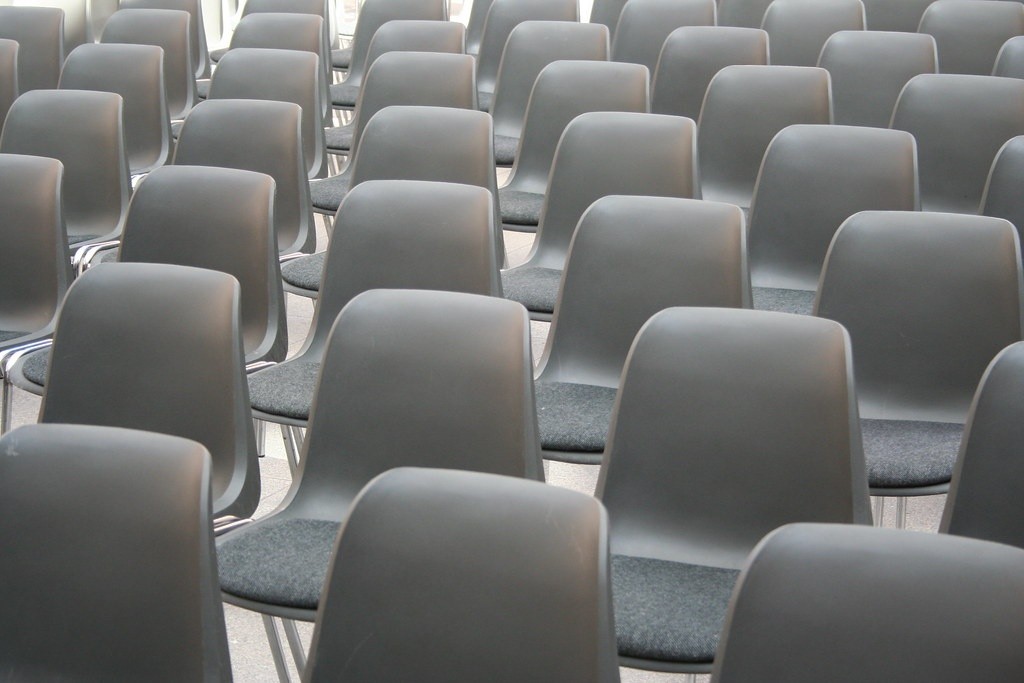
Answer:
[0,0,1024,683]
